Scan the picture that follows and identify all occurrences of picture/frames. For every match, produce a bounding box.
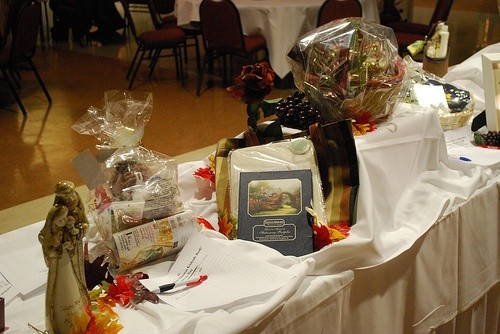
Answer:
[482,53,500,132]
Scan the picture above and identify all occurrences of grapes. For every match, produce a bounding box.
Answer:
[274,91,322,129]
[481,130,500,148]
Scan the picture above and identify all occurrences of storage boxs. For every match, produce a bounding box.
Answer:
[230,138,327,238]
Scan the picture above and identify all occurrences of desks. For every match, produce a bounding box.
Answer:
[0,44,500,334]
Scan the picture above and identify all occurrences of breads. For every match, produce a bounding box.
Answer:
[109,160,153,197]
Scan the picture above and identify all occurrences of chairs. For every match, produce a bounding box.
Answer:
[387,0,453,52]
[317,0,362,28]
[119,0,271,97]
[0,0,52,116]
